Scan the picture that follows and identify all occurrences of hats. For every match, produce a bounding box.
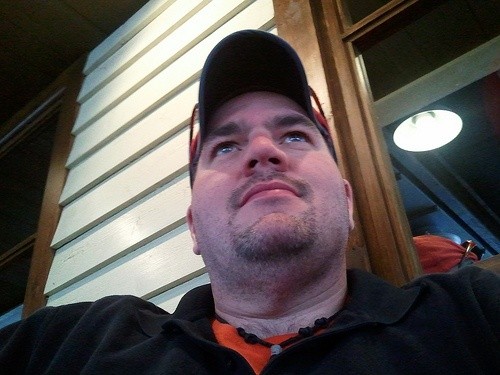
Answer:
[189,29,337,165]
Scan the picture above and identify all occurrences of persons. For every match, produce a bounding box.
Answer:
[0,28,500,375]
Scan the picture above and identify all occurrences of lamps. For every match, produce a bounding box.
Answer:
[390,106,463,154]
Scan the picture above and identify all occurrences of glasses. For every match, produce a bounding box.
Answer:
[189,85,326,141]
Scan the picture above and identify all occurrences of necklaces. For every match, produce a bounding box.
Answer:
[212,306,347,363]
